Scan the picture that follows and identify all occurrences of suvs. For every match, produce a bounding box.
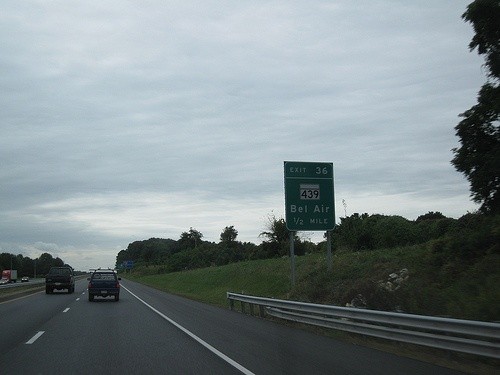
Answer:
[87,269,121,301]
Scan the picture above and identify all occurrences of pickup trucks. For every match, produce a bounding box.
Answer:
[45,266,75,293]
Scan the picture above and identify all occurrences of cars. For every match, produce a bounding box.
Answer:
[0,279,5,284]
[22,276,29,282]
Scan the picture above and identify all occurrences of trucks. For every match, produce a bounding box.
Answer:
[2,270,17,283]
